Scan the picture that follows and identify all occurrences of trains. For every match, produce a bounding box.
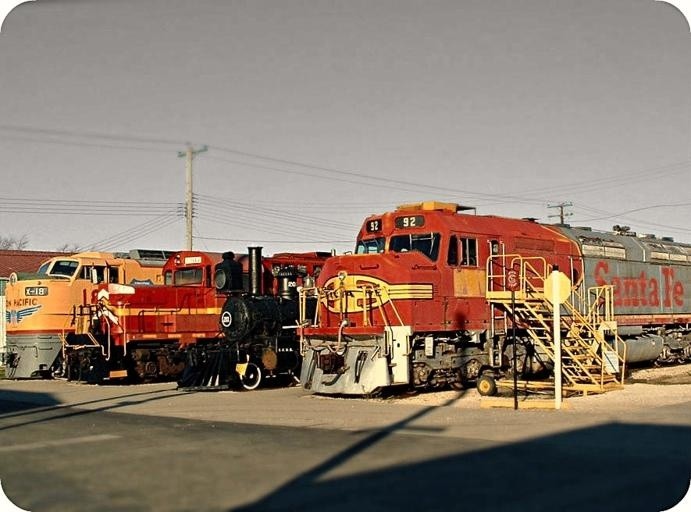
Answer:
[96,247,337,392]
[298,198,690,401]
[5,249,179,380]
